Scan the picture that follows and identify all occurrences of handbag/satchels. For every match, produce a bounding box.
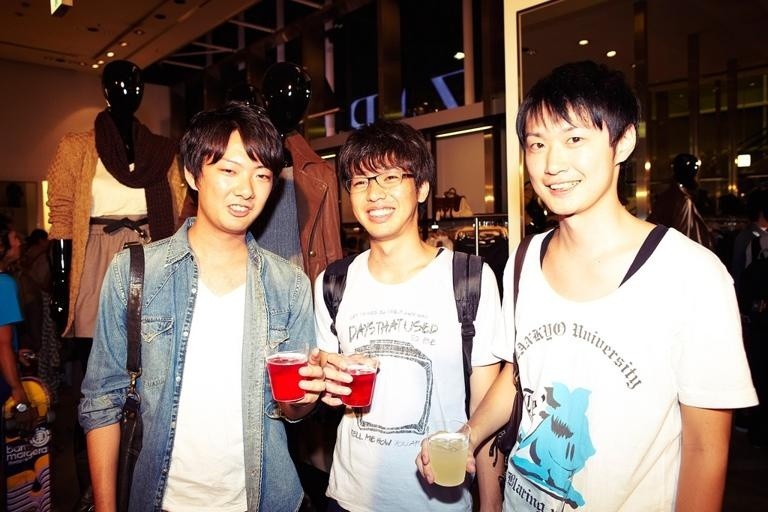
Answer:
[80,396,143,511]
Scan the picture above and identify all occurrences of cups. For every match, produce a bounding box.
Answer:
[424,417,472,489]
[261,340,311,404]
[338,352,380,410]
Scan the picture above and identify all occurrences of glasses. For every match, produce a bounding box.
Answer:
[345,166,415,194]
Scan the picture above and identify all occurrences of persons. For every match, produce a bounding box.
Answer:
[310,119,507,511]
[0,217,50,409]
[730,186,768,449]
[415,59,762,512]
[652,151,716,246]
[78,100,324,512]
